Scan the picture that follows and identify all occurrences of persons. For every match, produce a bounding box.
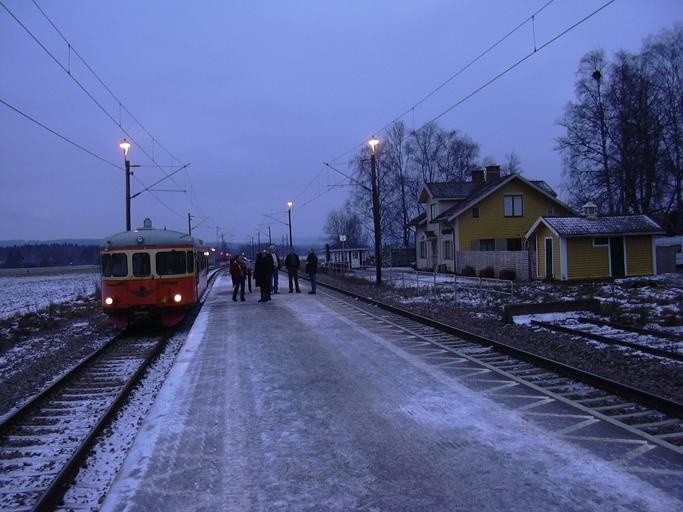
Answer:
[263,247,274,301]
[285,248,303,293]
[230,254,248,301]
[252,252,269,303]
[270,244,282,295]
[307,249,317,294]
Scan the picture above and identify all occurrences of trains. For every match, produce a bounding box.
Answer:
[100,227,207,328]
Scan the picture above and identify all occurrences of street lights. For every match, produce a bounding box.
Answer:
[287,201,292,248]
[120,138,130,232]
[366,137,381,288]
